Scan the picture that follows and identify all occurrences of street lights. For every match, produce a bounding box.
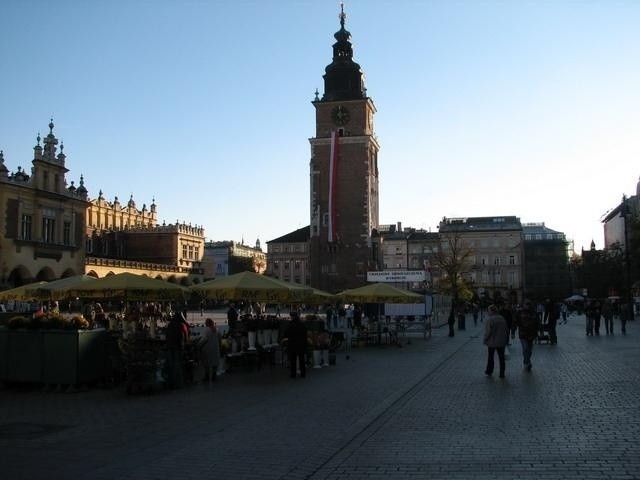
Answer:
[620,194,634,321]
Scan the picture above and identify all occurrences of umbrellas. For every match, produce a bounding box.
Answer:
[68,273,195,299]
[339,282,423,321]
[26,276,99,299]
[0,282,50,301]
[191,269,314,305]
[291,281,342,303]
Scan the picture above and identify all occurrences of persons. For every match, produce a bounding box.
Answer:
[444,299,561,378]
[225,299,365,388]
[560,299,633,337]
[1,299,220,354]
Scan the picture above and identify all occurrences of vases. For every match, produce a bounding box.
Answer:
[312,350,330,369]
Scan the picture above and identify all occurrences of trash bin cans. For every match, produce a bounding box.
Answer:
[328,349,336,365]
[458,314,465,330]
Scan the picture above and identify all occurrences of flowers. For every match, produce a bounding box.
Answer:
[307,330,332,350]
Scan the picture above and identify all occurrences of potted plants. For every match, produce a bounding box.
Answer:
[227,315,280,356]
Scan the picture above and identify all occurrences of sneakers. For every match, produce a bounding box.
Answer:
[499,369,506,379]
[484,371,493,378]
[585,328,626,336]
[287,364,308,378]
[202,378,217,386]
[523,362,533,370]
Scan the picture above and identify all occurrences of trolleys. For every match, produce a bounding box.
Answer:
[538,312,549,344]
